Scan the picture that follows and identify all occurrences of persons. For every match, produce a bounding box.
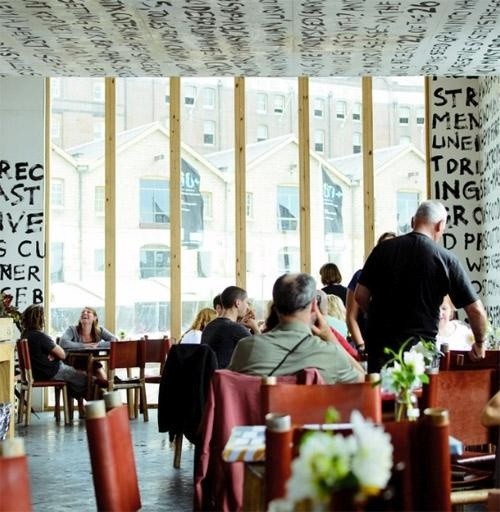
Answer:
[224,271,367,390]
[436,296,476,353]
[19,304,99,388]
[178,286,261,367]
[356,200,490,393]
[55,307,120,420]
[317,233,396,361]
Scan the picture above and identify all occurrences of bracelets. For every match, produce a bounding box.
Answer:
[475,338,488,345]
